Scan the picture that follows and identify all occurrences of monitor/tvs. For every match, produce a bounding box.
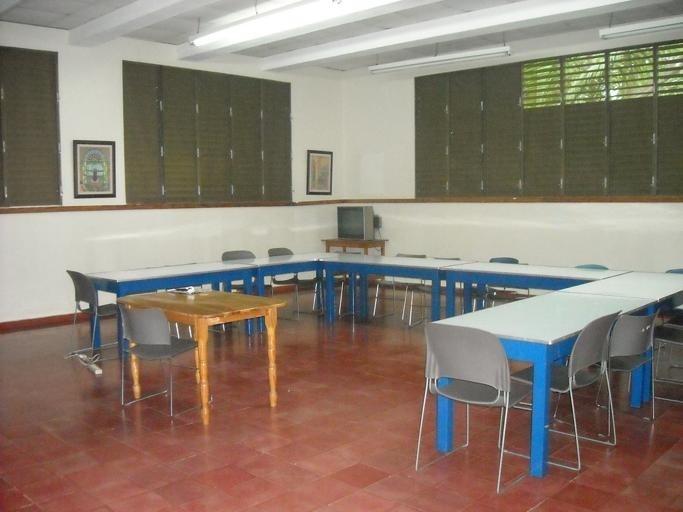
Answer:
[336,204,383,242]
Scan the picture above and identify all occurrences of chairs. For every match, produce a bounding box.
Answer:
[66,248,683,495]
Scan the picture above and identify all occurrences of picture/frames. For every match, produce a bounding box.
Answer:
[72,140,116,198]
[306,149,333,195]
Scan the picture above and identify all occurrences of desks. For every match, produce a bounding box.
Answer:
[321,238,389,309]
